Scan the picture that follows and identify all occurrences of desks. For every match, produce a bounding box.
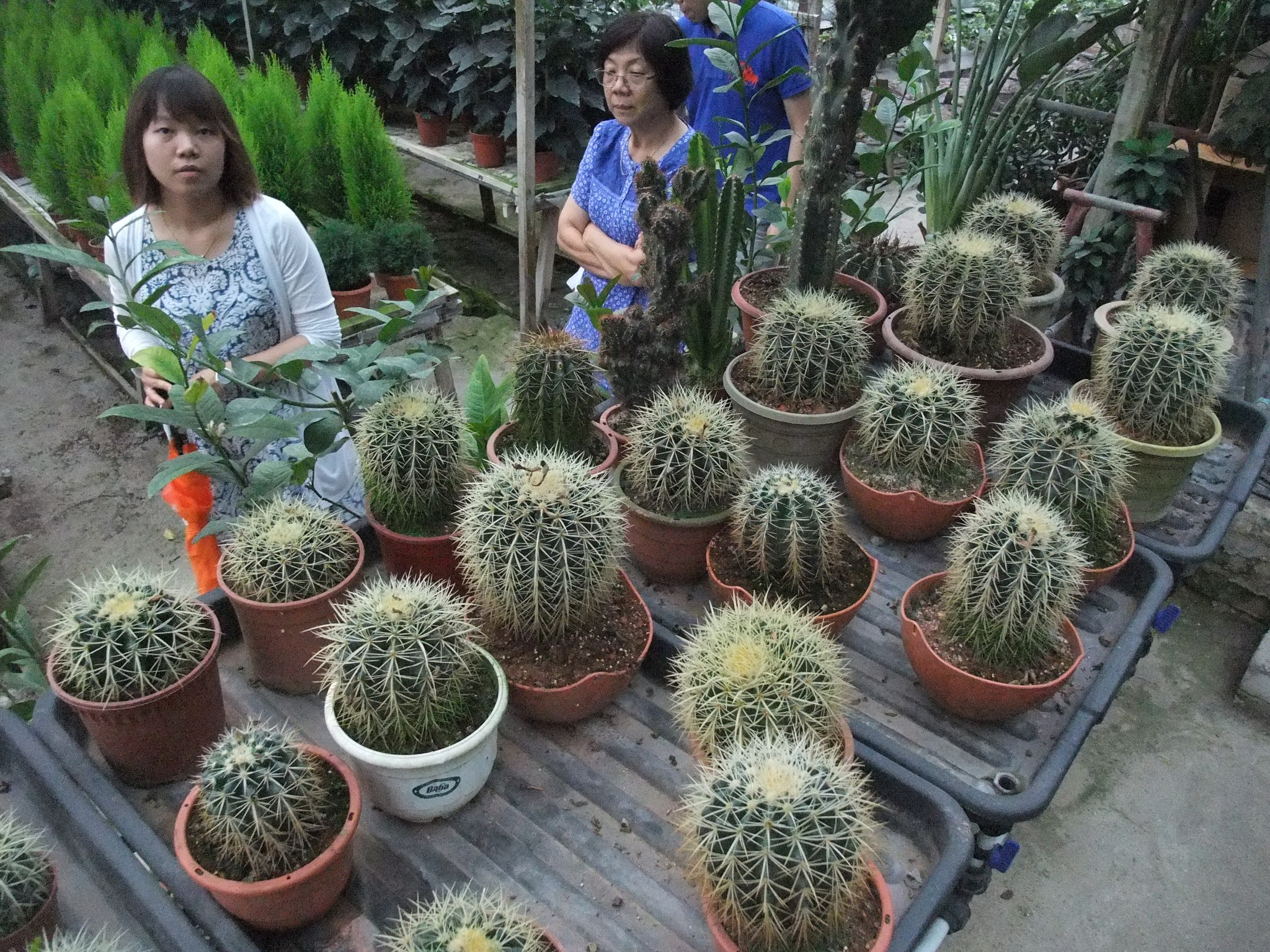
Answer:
[0,172,464,424]
[381,125,583,326]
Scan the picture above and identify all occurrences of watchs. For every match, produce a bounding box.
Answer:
[213,364,232,385]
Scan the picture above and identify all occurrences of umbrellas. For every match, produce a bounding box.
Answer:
[154,379,224,594]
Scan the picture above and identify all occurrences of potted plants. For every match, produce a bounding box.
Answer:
[0,0,1249,952]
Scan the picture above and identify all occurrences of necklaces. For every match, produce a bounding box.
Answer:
[160,206,214,258]
[620,133,662,176]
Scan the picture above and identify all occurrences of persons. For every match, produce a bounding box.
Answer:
[555,10,695,404]
[103,66,368,582]
[672,0,815,339]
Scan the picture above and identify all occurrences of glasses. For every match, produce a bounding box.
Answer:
[594,67,658,91]
